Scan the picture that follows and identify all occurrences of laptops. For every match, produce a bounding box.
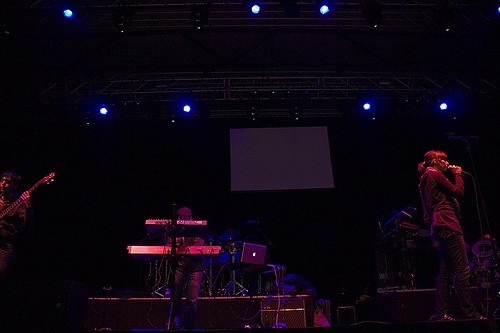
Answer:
[228,242,267,266]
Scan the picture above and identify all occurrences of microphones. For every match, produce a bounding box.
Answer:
[448,165,467,174]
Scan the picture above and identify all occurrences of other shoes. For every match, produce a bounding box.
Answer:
[466,312,487,319]
[435,313,456,321]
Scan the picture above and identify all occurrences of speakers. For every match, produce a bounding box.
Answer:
[260,298,312,328]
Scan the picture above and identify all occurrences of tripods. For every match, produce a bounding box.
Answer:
[202,242,248,295]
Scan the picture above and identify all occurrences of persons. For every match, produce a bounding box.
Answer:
[167,207,205,333]
[416,149,488,323]
[0,172,32,278]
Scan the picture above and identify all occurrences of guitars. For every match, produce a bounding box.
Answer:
[0,173,55,226]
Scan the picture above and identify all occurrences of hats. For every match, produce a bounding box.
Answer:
[179,207,192,216]
